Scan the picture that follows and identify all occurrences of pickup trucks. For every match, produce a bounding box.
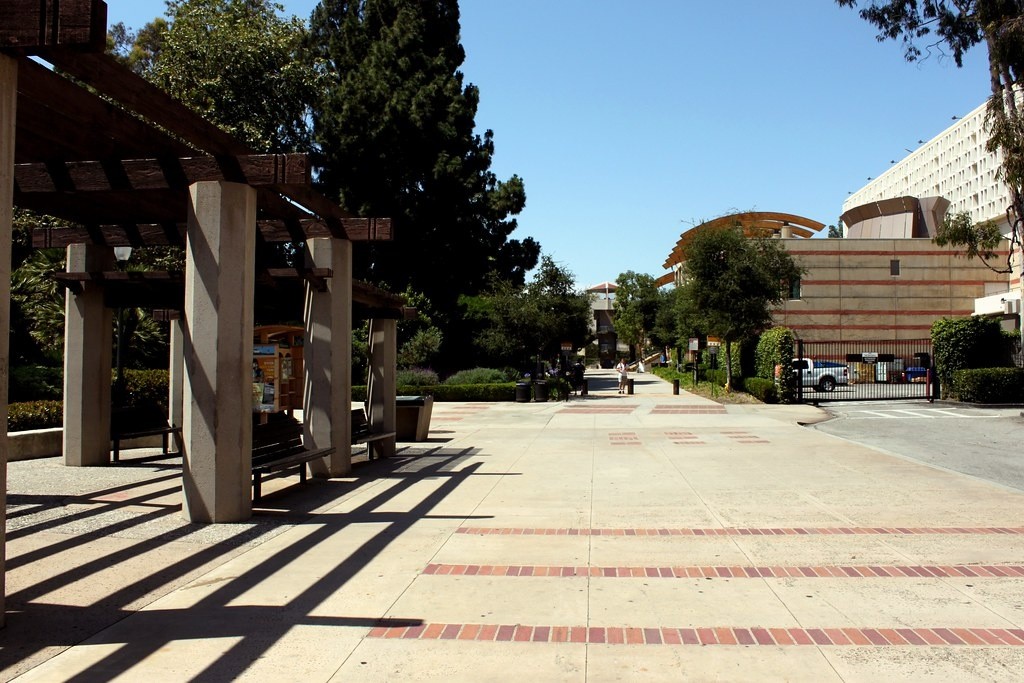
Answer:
[776,358,848,392]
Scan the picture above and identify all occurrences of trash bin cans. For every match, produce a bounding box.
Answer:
[533,379,549,402]
[516,382,531,402]
[364,395,434,441]
[906,367,926,382]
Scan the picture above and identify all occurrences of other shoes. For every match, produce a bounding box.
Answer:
[622,391,624,393]
[619,391,621,393]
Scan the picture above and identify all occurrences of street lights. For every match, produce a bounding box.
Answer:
[113,247,131,463]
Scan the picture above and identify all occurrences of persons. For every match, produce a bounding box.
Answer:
[616,358,629,394]
[252,358,264,383]
[573,358,585,396]
[660,353,665,363]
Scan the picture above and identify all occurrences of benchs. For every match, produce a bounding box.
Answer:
[110,426,182,463]
[252,423,336,501]
[349,409,396,460]
